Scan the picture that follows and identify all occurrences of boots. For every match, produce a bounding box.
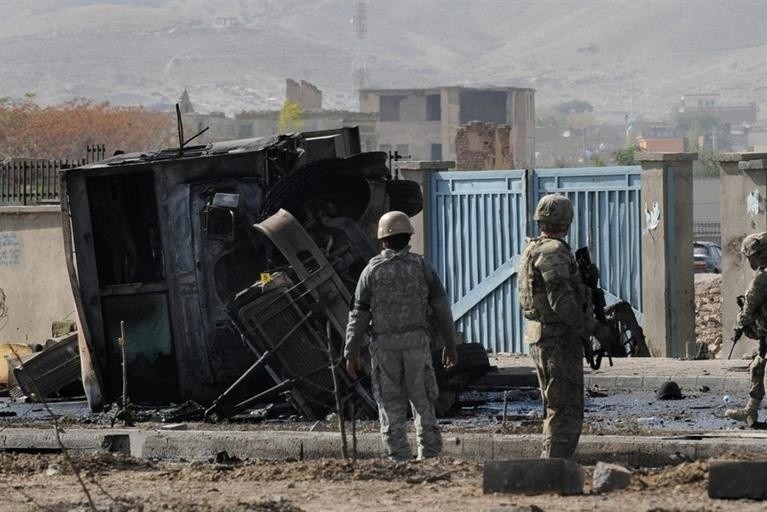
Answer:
[724,395,763,427]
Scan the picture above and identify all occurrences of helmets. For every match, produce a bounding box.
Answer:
[741,232,767,258]
[376,211,416,241]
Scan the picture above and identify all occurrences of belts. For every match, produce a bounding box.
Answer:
[374,323,422,335]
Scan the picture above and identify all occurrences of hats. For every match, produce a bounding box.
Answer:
[531,193,574,226]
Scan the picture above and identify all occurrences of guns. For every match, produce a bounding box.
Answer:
[575,247,612,370]
[728,295,744,360]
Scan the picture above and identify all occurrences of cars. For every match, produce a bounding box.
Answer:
[693,240,722,273]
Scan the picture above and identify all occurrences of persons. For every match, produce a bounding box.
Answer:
[343,211,459,462]
[723,230,766,429]
[517,194,614,462]
